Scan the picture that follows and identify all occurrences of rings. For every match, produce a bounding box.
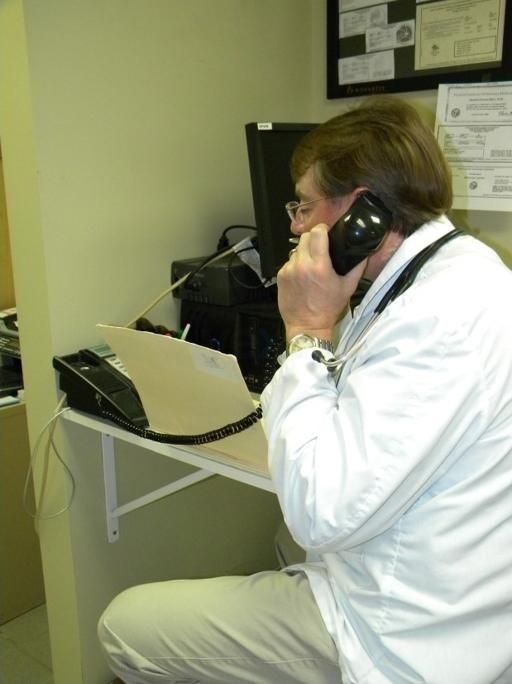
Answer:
[288,249,297,257]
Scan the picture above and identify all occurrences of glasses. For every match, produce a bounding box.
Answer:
[285,196,330,224]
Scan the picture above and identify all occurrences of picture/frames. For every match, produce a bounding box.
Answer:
[326,0,512,99]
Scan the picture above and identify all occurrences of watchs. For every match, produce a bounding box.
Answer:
[285,332,331,356]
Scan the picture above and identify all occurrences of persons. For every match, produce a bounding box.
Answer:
[95,98,511,684]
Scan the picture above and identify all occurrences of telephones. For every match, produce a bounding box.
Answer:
[328,190,393,275]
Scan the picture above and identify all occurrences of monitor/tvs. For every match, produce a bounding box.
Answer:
[245,122,322,278]
